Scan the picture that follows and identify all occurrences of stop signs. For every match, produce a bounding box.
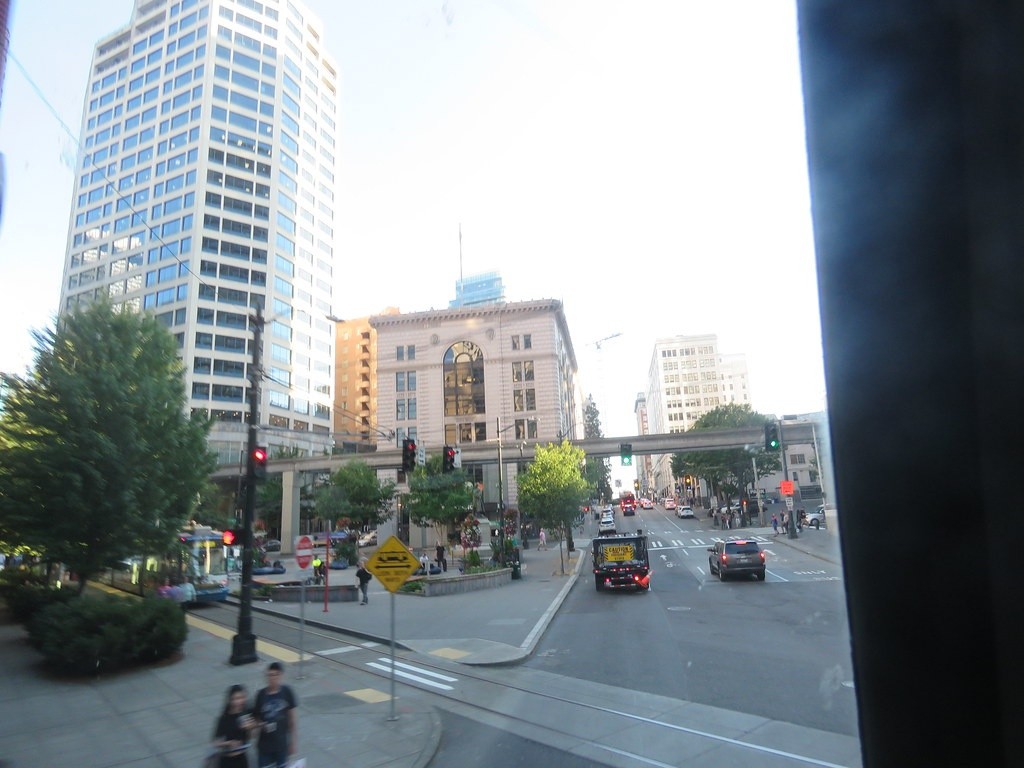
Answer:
[295,536,314,569]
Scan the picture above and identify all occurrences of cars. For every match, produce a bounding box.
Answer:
[357,529,377,548]
[620,491,653,517]
[264,540,281,552]
[602,503,616,521]
[805,504,825,526]
[597,518,616,535]
[708,496,778,518]
[659,497,694,519]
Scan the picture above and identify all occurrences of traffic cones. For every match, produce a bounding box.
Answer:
[637,569,655,589]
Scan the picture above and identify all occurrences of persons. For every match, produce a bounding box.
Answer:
[160,575,197,610]
[538,528,548,551]
[735,510,740,528]
[313,555,326,585]
[721,513,729,530]
[252,662,299,768]
[510,533,518,547]
[214,685,258,768]
[771,509,788,536]
[420,553,428,568]
[436,542,448,572]
[796,507,809,532]
[356,567,372,605]
[461,533,479,550]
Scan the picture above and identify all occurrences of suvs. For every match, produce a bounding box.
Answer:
[707,538,766,582]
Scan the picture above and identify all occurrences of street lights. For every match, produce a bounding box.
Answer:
[555,422,593,551]
[229,302,346,666]
[497,416,541,546]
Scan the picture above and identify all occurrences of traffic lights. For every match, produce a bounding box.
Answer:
[686,476,690,486]
[405,441,416,469]
[621,444,632,466]
[743,498,746,505]
[252,446,266,481]
[445,448,456,473]
[221,530,234,546]
[634,480,639,490]
[584,506,589,512]
[767,426,777,449]
[491,529,500,537]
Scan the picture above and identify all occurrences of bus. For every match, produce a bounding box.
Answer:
[127,520,229,606]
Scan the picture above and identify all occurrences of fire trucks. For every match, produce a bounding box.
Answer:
[590,529,652,592]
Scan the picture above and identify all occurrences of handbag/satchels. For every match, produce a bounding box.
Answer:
[203,752,224,768]
